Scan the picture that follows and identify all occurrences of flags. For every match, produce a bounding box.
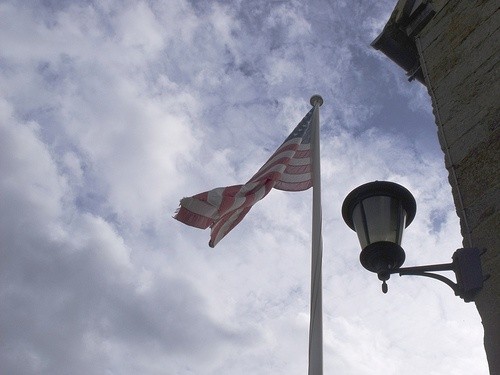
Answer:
[170,102,314,249]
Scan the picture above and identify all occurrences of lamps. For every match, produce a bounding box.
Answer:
[341,180,488,303]
